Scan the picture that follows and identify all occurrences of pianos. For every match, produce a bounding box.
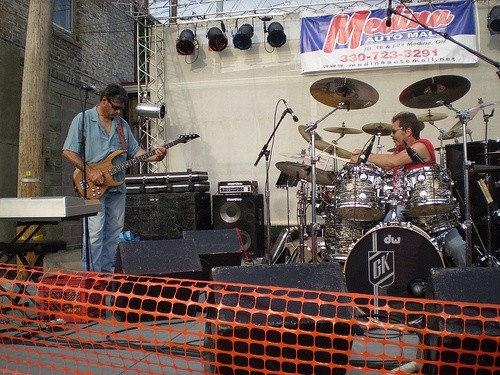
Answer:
[0,196,102,317]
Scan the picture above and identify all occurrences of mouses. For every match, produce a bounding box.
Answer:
[412,282,428,296]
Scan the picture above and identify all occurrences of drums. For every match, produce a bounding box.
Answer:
[341,222,446,332]
[409,211,460,232]
[333,166,385,222]
[322,208,366,262]
[405,164,455,216]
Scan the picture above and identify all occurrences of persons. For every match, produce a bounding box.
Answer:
[353,112,466,267]
[62,85,167,291]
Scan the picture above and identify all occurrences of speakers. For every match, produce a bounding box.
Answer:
[111,194,264,323]
[446,139,499,266]
[203,261,356,375]
[416,266,500,375]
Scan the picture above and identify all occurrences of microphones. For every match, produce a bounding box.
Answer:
[282,100,299,123]
[386,0,392,27]
[406,145,425,165]
[80,85,97,91]
[357,137,375,164]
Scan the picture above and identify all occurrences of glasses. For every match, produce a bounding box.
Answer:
[107,99,124,111]
[391,126,406,134]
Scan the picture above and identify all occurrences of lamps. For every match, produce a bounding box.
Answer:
[487,5,500,36]
[258,15,286,53]
[136,102,166,120]
[233,16,256,50]
[206,20,228,51]
[176,23,199,64]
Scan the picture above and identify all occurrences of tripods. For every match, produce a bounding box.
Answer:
[473,108,500,267]
[285,175,325,265]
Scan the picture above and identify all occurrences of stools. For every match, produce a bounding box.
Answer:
[0,240,66,324]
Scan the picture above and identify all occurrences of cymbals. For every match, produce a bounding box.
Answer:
[362,123,395,136]
[324,126,363,136]
[415,112,448,121]
[309,77,379,109]
[398,74,471,109]
[438,128,472,140]
[297,124,322,145]
[313,140,354,159]
[274,161,332,186]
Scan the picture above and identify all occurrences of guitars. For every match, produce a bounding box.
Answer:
[73,132,200,199]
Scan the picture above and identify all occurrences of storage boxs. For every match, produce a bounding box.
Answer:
[37,275,106,323]
